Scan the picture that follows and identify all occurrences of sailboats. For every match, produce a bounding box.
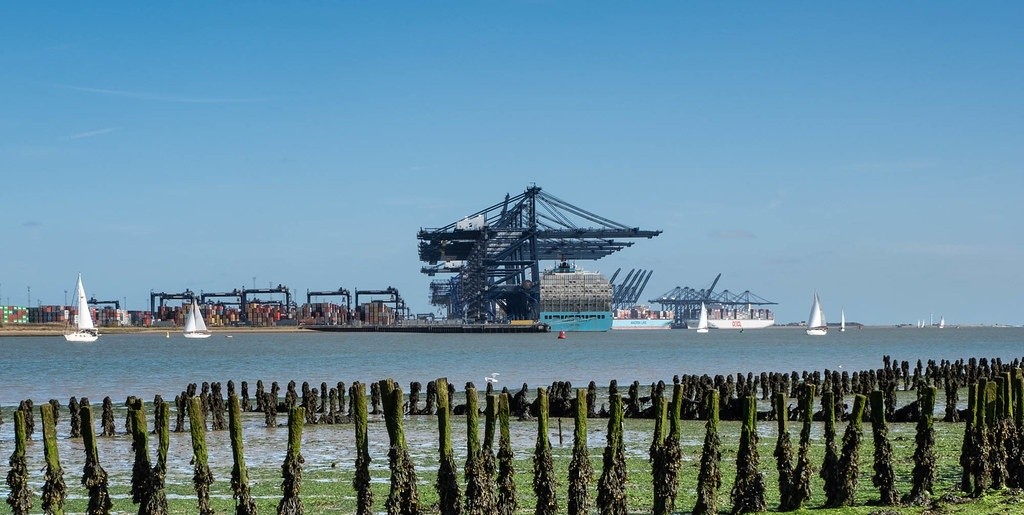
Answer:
[695,302,709,333]
[62,273,102,344]
[181,299,214,338]
[806,292,828,336]
[840,307,845,332]
[938,315,945,329]
[917,319,926,328]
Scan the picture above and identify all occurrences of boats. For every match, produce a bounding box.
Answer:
[685,305,776,330]
[532,258,614,333]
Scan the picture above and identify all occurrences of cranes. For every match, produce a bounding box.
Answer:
[414,182,782,320]
